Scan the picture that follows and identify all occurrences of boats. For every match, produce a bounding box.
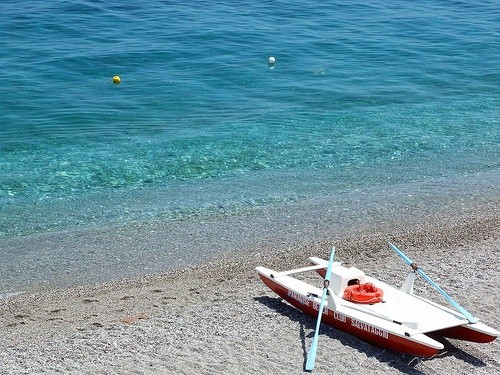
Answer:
[255,254,500,361]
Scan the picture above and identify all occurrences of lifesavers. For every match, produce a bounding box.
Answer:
[344,283,383,304]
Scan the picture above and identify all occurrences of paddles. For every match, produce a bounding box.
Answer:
[303,245,335,370]
[388,243,478,325]
